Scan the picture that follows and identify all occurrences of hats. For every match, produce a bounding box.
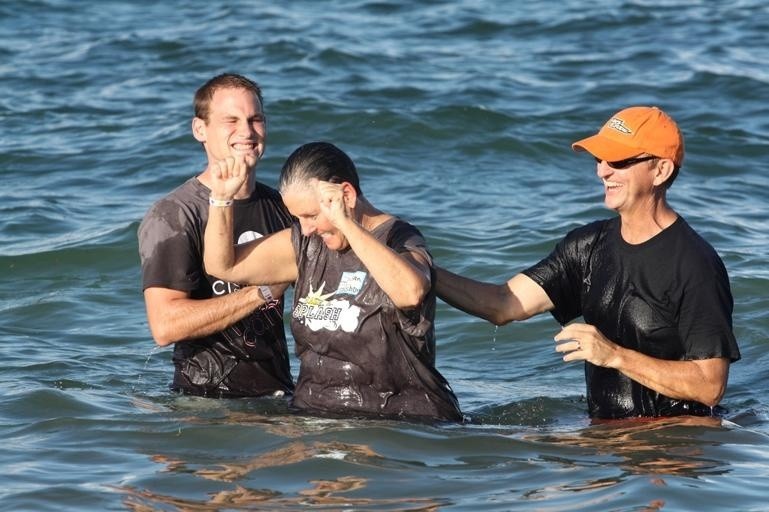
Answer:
[571,104,683,167]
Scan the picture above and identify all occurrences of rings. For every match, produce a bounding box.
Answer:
[575,340,581,351]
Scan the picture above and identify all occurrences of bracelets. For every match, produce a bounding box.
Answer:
[210,195,235,207]
[260,286,273,303]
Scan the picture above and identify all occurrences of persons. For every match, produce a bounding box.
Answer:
[434,106,740,419]
[204,142,461,422]
[137,74,298,399]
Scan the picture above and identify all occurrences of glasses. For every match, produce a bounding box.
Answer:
[592,154,655,168]
